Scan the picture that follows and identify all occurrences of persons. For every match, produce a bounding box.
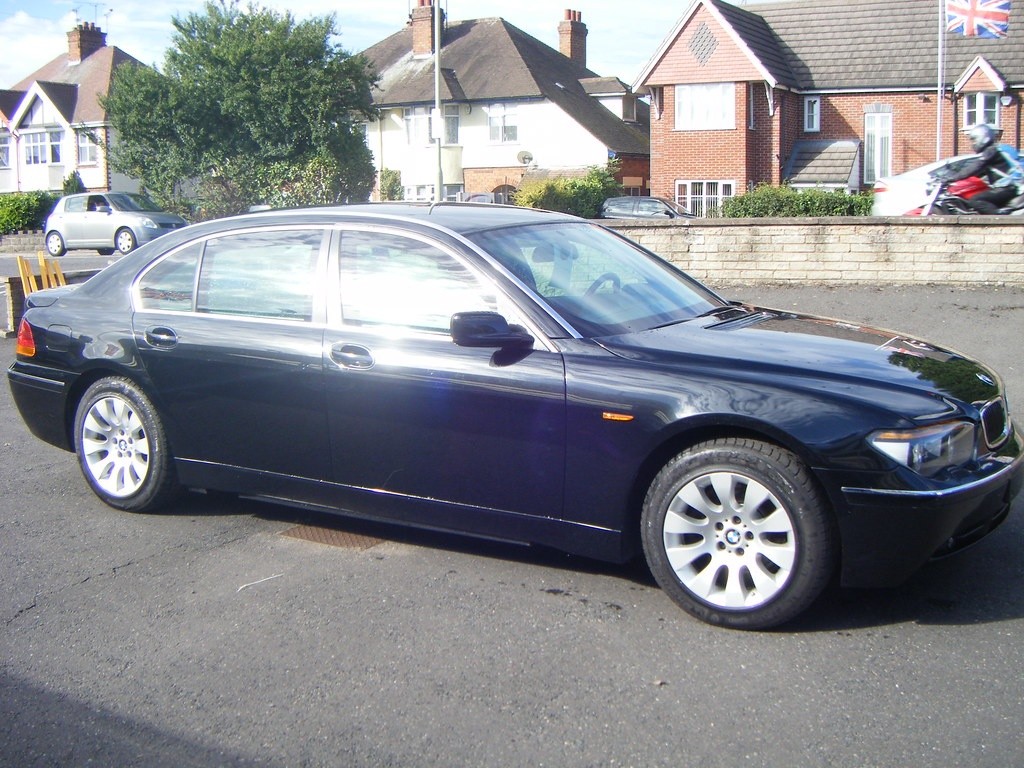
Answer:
[940,125,1023,217]
[96,201,105,207]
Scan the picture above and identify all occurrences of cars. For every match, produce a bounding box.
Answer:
[6,202,1024,631]
[867,149,1023,217]
[44,191,191,256]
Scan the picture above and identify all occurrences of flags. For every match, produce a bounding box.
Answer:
[942,0,1011,40]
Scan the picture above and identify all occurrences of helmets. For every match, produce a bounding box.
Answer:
[970,124,995,153]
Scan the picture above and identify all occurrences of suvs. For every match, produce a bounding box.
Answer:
[600,196,698,219]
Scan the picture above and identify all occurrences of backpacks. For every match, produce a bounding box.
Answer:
[986,143,1024,195]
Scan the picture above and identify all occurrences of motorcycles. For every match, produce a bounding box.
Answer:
[902,158,1023,218]
